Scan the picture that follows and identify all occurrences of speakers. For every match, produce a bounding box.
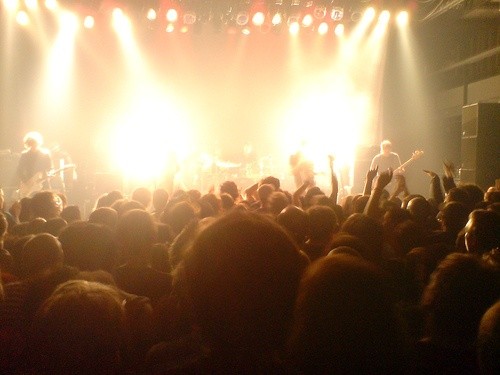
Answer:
[460,103,500,192]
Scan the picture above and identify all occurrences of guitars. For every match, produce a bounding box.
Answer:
[19,164,75,198]
[393,151,424,176]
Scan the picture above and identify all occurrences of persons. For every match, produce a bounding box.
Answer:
[0,132,500,375]
